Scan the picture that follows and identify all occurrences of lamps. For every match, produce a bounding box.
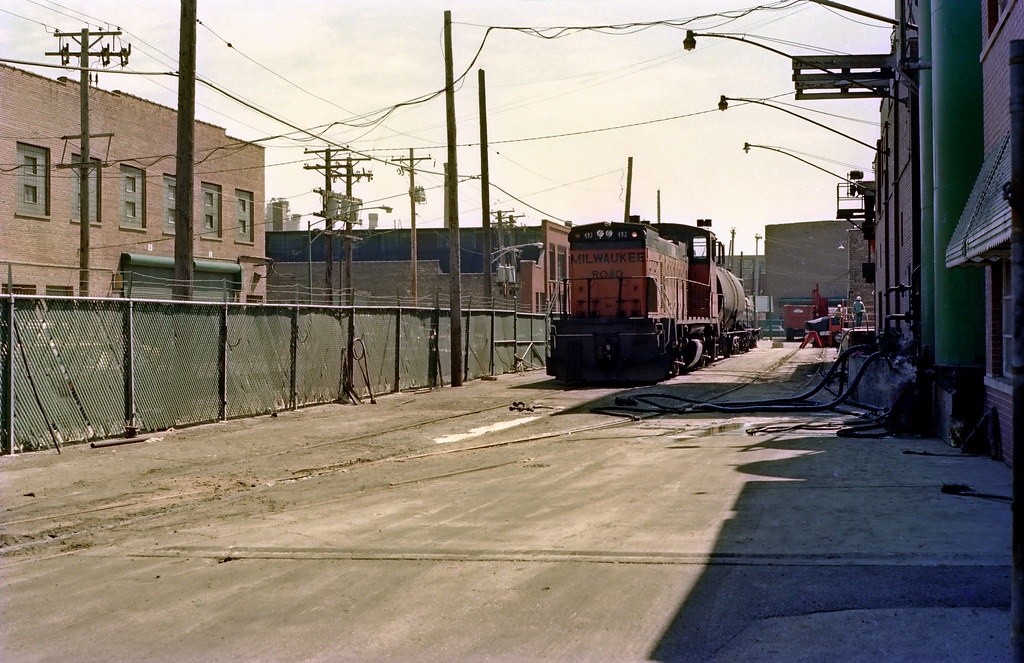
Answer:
[718,95,890,159]
[743,142,876,194]
[683,30,907,108]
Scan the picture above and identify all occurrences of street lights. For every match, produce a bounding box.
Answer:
[482,240,544,312]
[754,233,762,263]
[307,205,394,306]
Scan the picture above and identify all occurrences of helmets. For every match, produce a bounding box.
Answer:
[856,296,861,300]
[838,305,841,308]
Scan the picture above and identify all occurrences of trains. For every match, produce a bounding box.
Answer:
[538,213,765,382]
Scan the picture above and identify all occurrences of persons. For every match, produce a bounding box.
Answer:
[835,304,843,325]
[853,296,866,327]
